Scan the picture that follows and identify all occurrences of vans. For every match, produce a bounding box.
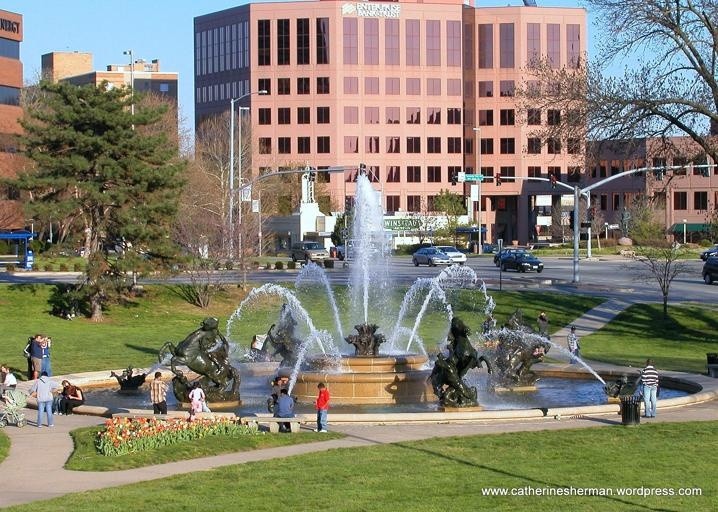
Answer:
[290,240,330,263]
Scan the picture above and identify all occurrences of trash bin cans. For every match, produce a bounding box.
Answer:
[619,395,641,427]
[706,353,718,378]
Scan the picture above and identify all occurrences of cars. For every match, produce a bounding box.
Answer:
[701,255,718,285]
[500,251,545,274]
[412,247,453,267]
[78,247,91,257]
[336,239,359,261]
[433,245,468,265]
[700,244,718,261]
[493,247,526,267]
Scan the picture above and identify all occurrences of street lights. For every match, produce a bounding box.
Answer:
[682,218,689,244]
[123,49,146,129]
[228,87,268,260]
[236,104,251,258]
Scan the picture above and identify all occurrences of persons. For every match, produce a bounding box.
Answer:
[189,382,205,412]
[480,307,549,337]
[641,359,659,418]
[0,335,86,428]
[567,326,579,363]
[314,383,331,432]
[150,372,169,414]
[273,389,294,432]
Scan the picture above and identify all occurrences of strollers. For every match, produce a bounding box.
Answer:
[0,390,29,430]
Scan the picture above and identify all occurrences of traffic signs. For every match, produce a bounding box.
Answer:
[465,174,484,181]
[457,172,466,182]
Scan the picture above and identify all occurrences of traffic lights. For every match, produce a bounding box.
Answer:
[568,209,574,230]
[495,172,502,186]
[580,222,591,228]
[359,162,368,179]
[588,207,596,222]
[310,168,317,183]
[550,174,557,192]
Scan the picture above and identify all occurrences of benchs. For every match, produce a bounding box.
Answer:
[113,412,189,422]
[191,413,235,422]
[241,416,301,433]
[26,399,109,416]
